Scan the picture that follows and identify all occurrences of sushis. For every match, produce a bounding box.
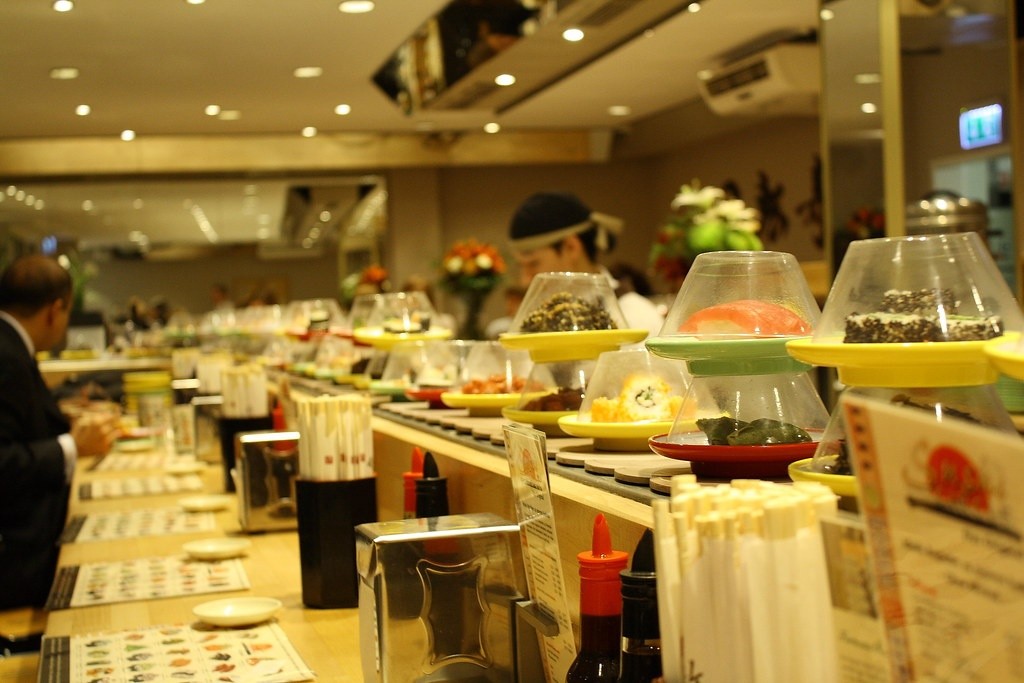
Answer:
[383,310,431,336]
[593,373,688,423]
[677,298,812,335]
[843,288,1003,343]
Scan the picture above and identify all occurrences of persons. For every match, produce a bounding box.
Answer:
[380,274,456,383]
[113,282,280,348]
[502,192,668,354]
[0,256,132,657]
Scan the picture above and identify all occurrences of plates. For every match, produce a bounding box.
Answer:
[192,596,281,626]
[284,365,371,389]
[787,453,857,509]
[501,403,585,439]
[59,347,96,359]
[178,495,231,512]
[558,409,730,450]
[163,462,209,474]
[122,372,174,415]
[784,332,1022,388]
[404,386,458,410]
[354,326,453,351]
[440,386,561,416]
[499,328,649,363]
[183,537,251,561]
[646,335,815,379]
[367,379,412,401]
[984,331,1024,383]
[647,426,825,481]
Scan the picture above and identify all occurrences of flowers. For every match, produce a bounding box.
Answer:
[669,177,764,254]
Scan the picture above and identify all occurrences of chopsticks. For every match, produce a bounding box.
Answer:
[651,471,840,683]
[297,392,375,481]
[196,355,233,393]
[220,363,269,419]
[172,346,201,380]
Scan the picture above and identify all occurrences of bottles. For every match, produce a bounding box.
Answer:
[416,450,450,520]
[402,446,425,519]
[614,525,663,683]
[565,513,630,683]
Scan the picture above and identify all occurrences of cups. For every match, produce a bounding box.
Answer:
[218,412,275,491]
[172,403,196,452]
[295,478,378,608]
[137,393,166,428]
